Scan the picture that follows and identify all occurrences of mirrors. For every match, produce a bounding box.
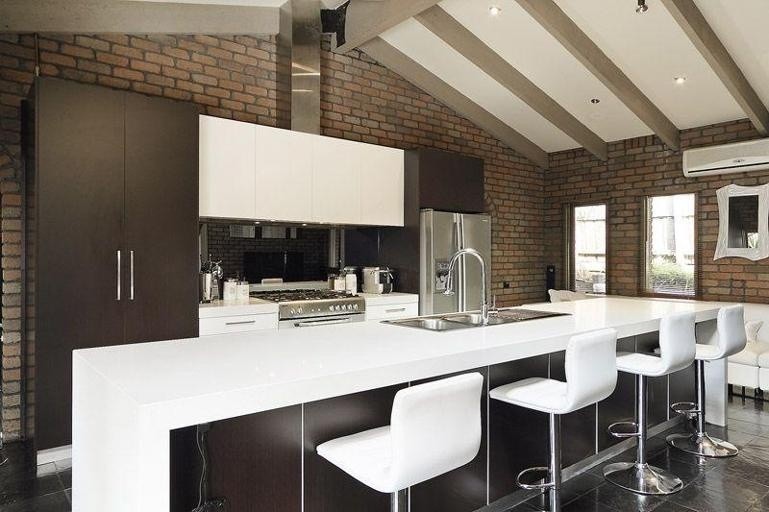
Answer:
[712,182,769,262]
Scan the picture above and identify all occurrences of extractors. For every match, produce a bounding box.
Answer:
[200,1,404,230]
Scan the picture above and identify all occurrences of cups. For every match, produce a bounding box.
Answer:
[199,270,214,304]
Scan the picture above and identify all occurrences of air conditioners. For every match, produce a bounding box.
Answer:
[682,138,769,180]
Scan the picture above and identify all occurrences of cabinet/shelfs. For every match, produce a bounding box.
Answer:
[358,292,419,321]
[199,297,279,335]
[198,115,405,228]
[404,147,485,214]
[21,73,200,480]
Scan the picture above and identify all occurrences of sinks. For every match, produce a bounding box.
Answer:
[379,315,482,333]
[442,310,519,326]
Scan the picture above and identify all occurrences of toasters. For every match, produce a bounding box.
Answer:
[360,265,393,294]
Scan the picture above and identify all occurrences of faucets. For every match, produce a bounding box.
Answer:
[442,247,487,318]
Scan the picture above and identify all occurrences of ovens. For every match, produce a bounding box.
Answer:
[278,312,366,332]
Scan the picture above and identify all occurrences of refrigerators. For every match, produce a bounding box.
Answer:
[421,208,491,317]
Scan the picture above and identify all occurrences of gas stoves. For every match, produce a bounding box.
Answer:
[247,289,357,302]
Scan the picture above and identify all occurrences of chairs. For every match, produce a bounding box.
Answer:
[601,311,695,498]
[728,302,769,407]
[667,304,747,460]
[315,371,484,512]
[489,327,618,512]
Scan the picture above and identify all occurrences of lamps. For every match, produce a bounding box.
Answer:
[590,98,600,108]
[636,0,649,15]
[488,5,501,18]
[672,72,689,85]
